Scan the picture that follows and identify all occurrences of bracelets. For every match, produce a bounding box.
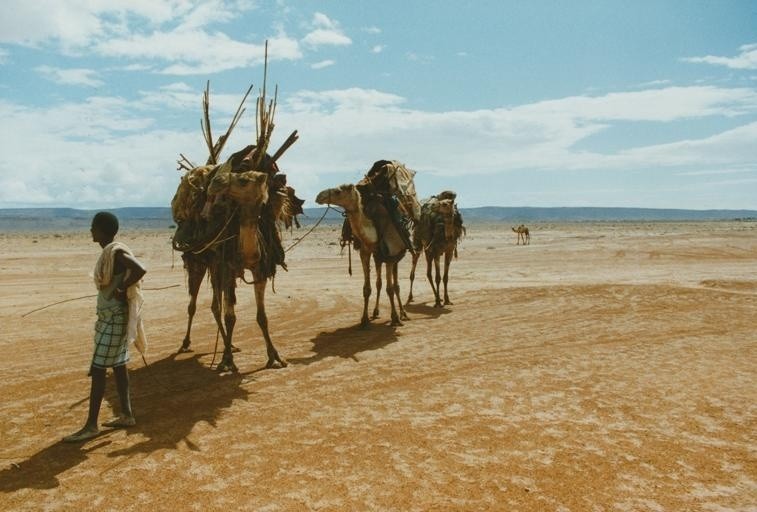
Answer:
[116,287,126,293]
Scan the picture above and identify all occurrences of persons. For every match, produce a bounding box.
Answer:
[62,211,148,443]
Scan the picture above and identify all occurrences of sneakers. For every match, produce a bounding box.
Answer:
[102,413,136,427]
[62,431,100,442]
